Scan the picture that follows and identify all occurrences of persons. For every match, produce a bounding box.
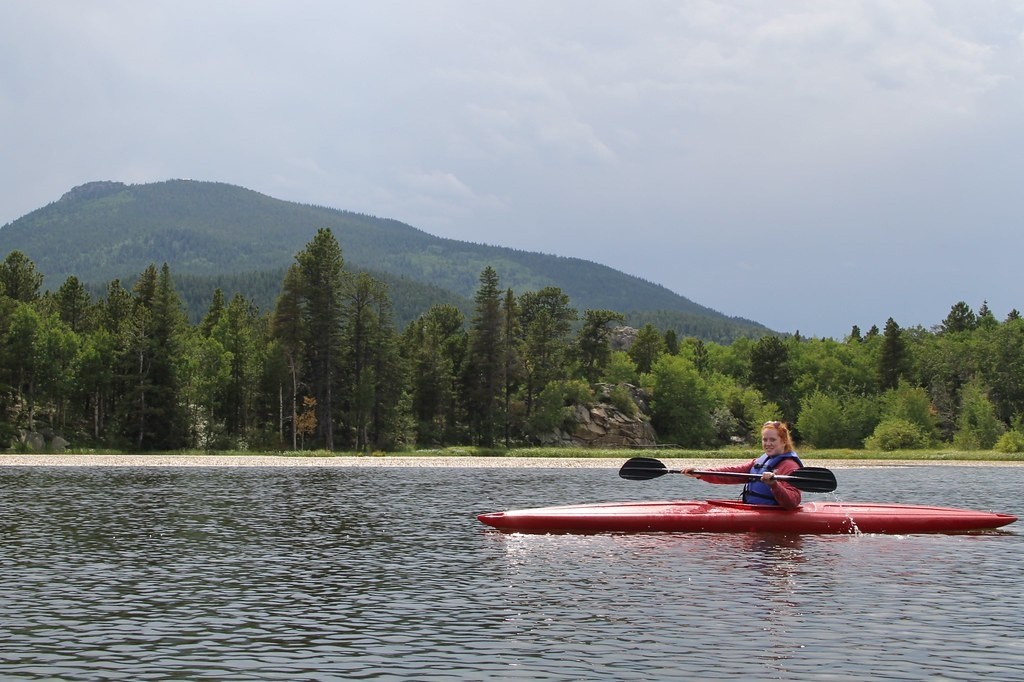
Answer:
[681,419,806,508]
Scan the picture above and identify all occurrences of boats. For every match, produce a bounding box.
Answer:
[479,497,1024,536]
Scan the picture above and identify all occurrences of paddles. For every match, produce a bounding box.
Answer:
[619,457,837,493]
